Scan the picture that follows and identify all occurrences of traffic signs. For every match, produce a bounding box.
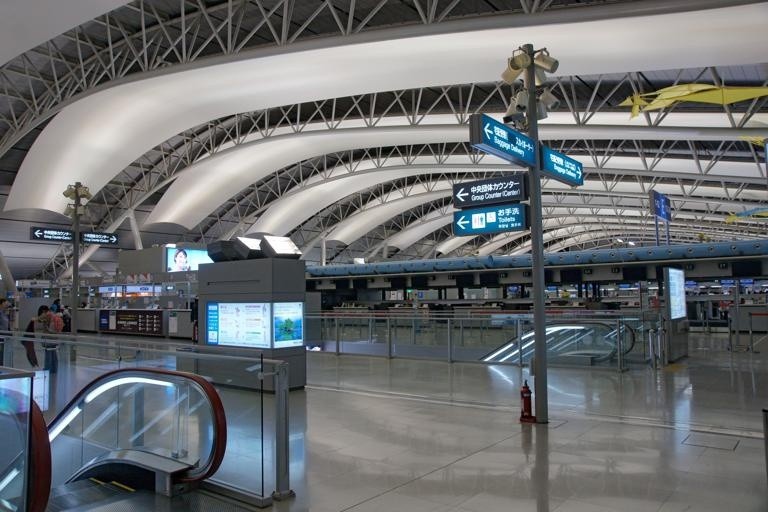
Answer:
[451,207,530,235]
[472,113,534,167]
[31,227,76,242]
[449,175,528,206]
[82,233,119,245]
[541,147,586,187]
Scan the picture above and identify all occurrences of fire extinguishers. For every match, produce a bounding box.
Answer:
[520,380,535,422]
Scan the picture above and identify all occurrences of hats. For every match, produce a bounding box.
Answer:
[50,304,57,311]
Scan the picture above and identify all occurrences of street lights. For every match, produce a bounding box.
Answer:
[64,182,92,362]
[498,42,560,425]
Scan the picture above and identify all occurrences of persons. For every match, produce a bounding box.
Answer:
[0,298,11,367]
[584,296,602,310]
[30,304,64,375]
[48,299,87,333]
[168,249,192,273]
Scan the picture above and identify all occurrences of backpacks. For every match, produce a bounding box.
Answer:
[53,316,64,332]
